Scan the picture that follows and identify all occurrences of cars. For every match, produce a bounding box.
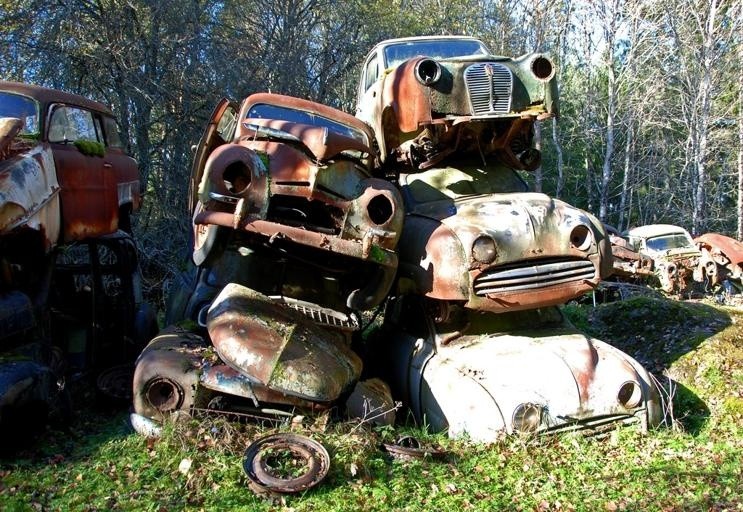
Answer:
[355,38,564,175]
[602,220,743,296]
[0,77,145,252]
[402,322,667,455]
[396,166,616,320]
[133,269,392,453]
[1,232,164,434]
[182,94,404,306]
